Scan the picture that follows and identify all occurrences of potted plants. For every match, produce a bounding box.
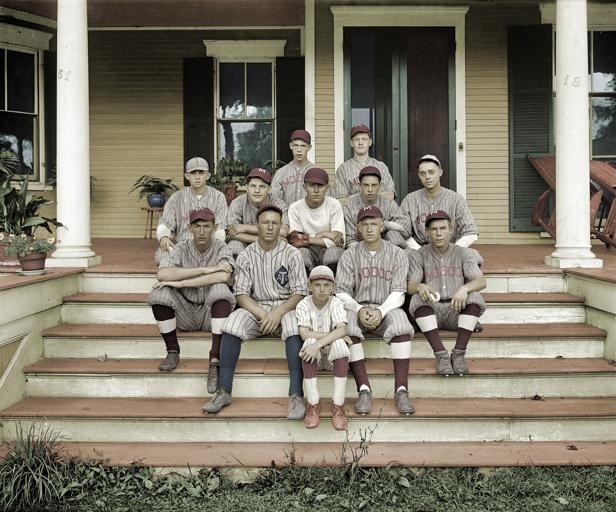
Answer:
[216,154,250,206]
[0,169,69,262]
[128,174,181,208]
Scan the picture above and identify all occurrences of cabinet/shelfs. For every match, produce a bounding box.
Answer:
[525,150,616,249]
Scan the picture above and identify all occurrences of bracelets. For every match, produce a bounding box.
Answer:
[417,283,425,291]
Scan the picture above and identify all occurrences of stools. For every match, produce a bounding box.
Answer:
[140,206,164,239]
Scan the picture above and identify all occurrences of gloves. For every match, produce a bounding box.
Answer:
[287,229,311,249]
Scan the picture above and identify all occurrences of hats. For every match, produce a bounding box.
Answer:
[350,124,369,139]
[304,168,328,185]
[417,154,441,169]
[358,166,381,183]
[309,265,334,281]
[425,210,450,225]
[256,205,283,219]
[289,130,311,144]
[357,205,382,223]
[248,167,272,183]
[185,157,209,172]
[189,207,215,224]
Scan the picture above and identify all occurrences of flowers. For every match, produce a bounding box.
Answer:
[0,230,61,258]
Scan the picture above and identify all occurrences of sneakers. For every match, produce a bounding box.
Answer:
[354,389,372,414]
[330,401,348,431]
[159,350,180,373]
[394,389,416,414]
[287,393,321,429]
[206,361,220,394]
[434,350,469,376]
[201,388,232,413]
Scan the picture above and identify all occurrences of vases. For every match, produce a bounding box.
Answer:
[16,252,47,271]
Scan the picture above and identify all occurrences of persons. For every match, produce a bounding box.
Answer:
[336,124,397,203]
[202,203,309,420]
[228,168,290,258]
[294,265,350,431]
[271,129,315,205]
[335,205,416,414]
[344,165,412,249]
[146,207,234,393]
[155,157,229,264]
[287,167,346,280]
[400,154,483,270]
